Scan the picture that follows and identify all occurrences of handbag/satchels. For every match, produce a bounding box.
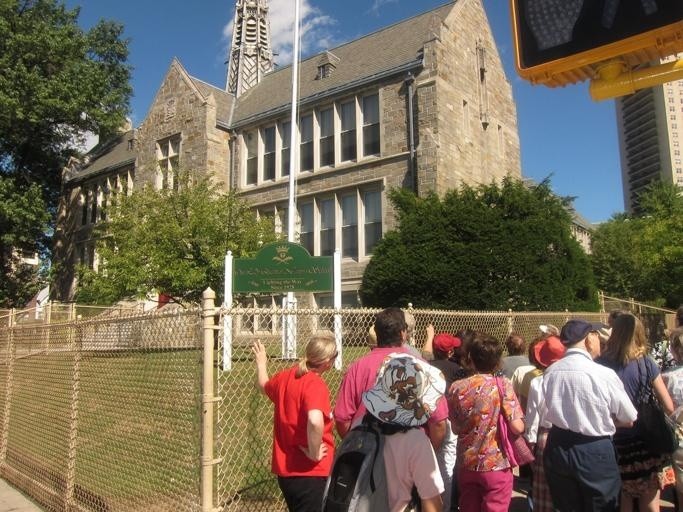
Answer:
[495,411,536,467]
[640,396,679,453]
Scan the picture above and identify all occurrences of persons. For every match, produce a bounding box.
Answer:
[448,334,525,512]
[322,353,447,512]
[510,335,547,401]
[656,328,683,512]
[426,333,466,512]
[519,337,565,512]
[501,335,529,380]
[334,308,449,512]
[525,321,638,512]
[676,306,683,327]
[423,324,475,383]
[252,337,338,512]
[594,309,676,512]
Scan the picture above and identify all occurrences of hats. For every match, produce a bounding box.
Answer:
[432,333,461,352]
[535,336,566,368]
[560,319,604,345]
[361,352,446,426]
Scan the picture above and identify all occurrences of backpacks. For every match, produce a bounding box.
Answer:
[322,413,389,512]
